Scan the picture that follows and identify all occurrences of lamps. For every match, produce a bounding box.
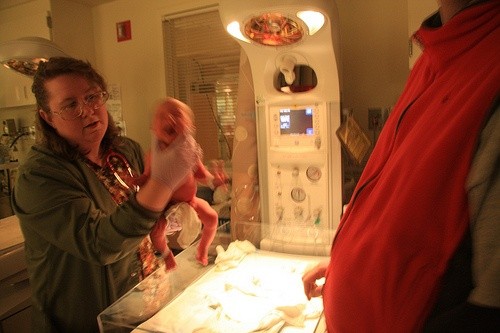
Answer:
[0,35,72,80]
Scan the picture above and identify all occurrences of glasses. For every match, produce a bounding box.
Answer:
[49,91,110,121]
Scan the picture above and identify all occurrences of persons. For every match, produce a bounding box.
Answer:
[300,0,500,333]
[11,56,229,333]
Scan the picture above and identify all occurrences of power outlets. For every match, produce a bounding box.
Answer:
[29,126,37,140]
